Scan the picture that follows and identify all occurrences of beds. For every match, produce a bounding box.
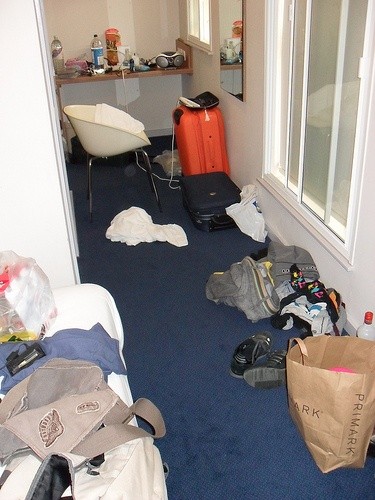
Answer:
[0,284,171,500]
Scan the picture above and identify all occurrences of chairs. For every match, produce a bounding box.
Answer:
[64,102,162,214]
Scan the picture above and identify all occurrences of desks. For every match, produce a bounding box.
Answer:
[53,63,192,135]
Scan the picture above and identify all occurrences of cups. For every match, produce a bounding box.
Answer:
[51,35,65,74]
[225,48,236,60]
[91,33,104,68]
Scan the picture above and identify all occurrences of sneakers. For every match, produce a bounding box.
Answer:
[230,331,274,378]
[244,351,286,388]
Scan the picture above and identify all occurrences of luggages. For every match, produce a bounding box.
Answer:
[173,105,230,176]
[183,171,244,232]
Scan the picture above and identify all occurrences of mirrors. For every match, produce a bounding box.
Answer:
[218,0,246,103]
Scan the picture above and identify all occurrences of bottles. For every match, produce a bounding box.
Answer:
[129,58,135,72]
[356,311,375,342]
[232,21,242,54]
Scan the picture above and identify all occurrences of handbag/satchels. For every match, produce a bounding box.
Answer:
[285,333,375,474]
[179,92,220,109]
[0,358,166,467]
[226,184,269,243]
